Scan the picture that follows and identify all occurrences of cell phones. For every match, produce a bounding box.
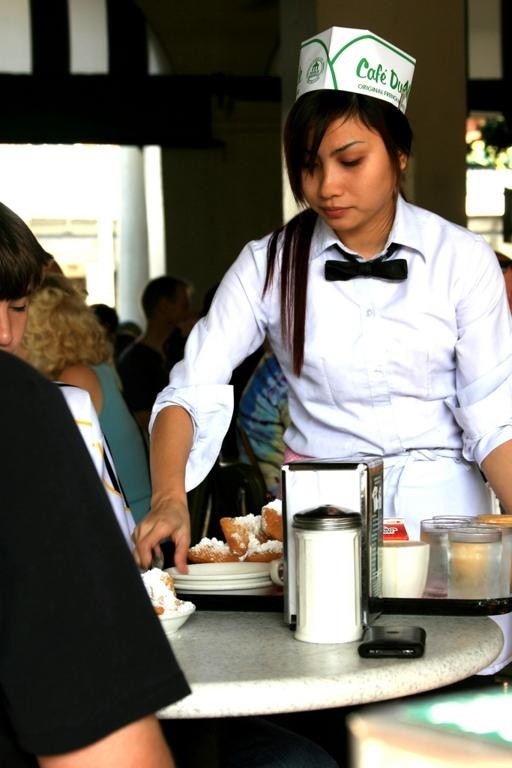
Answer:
[358,625,426,659]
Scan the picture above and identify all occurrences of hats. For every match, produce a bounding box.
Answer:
[295,25,417,115]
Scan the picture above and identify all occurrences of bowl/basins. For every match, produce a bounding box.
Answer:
[158,604,196,635]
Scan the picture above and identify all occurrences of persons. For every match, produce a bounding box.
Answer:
[1,204,288,572]
[130,89,512,577]
[0,350,191,768]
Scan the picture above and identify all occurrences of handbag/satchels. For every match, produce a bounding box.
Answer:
[187,463,267,547]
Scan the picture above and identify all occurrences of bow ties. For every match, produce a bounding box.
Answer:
[325,243,407,280]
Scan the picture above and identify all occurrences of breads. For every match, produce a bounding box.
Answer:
[186,495,282,564]
[133,567,190,615]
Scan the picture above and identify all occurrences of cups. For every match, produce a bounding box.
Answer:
[376,538,433,600]
[419,515,510,599]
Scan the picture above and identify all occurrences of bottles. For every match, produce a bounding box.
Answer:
[290,501,366,645]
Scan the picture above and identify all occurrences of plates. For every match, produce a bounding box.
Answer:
[166,556,273,593]
